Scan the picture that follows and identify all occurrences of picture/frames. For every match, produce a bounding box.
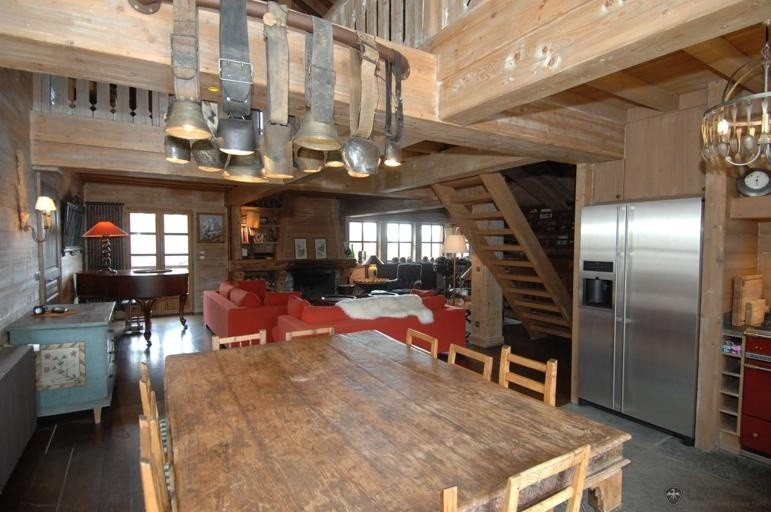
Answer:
[258,216,268,225]
[291,237,307,260]
[196,211,228,246]
[312,237,327,260]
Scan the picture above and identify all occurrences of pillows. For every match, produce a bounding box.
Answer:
[263,289,302,307]
[238,279,266,303]
[407,288,432,298]
[284,294,312,318]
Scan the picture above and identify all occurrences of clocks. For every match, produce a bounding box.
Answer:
[736,168,770,199]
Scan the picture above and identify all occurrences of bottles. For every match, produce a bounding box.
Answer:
[350,243,355,258]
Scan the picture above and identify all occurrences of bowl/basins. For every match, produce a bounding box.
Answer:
[338,284,355,295]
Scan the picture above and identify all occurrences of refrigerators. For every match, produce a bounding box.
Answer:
[577,193,704,447]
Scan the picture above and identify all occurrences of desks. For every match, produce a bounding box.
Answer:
[442,300,473,340]
[353,278,386,292]
[159,328,636,512]
[304,293,369,306]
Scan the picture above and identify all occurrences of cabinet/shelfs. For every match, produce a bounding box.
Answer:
[238,205,282,263]
[737,328,771,466]
[4,301,118,426]
[716,328,748,453]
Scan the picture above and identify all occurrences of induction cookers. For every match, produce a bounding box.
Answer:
[745,320,771,361]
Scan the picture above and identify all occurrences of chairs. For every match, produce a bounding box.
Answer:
[404,327,441,358]
[384,262,423,292]
[208,330,268,351]
[135,357,173,512]
[283,327,335,343]
[497,346,560,408]
[446,341,496,379]
[501,443,593,512]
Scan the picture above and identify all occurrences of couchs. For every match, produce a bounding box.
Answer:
[201,280,304,349]
[269,288,467,356]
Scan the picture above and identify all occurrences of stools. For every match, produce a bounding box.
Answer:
[120,299,146,337]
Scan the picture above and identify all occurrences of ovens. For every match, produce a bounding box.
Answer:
[742,359,771,463]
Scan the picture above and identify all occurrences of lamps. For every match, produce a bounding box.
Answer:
[442,234,468,308]
[18,194,58,248]
[81,221,130,274]
[360,254,384,281]
[697,19,769,172]
